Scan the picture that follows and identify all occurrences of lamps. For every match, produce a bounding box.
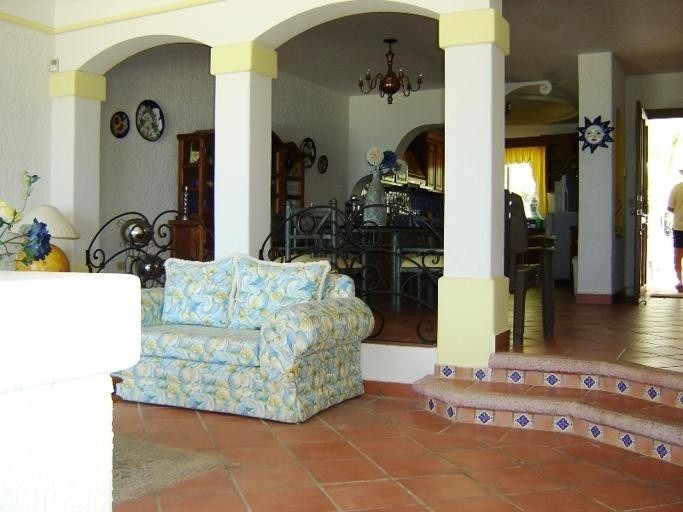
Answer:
[6,204,79,271]
[359,38,423,104]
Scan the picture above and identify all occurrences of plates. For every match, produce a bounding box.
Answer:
[299,136,328,174]
[110,99,165,142]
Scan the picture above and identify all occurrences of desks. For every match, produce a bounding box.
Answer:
[325,225,423,313]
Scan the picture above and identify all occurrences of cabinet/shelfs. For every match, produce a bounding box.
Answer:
[169,129,305,261]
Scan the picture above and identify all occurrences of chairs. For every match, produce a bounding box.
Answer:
[397,247,442,313]
[283,199,361,303]
[503,188,556,347]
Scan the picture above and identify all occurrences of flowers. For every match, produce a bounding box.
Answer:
[0,170,52,271]
[366,146,409,176]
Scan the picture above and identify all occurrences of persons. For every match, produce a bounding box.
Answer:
[667,168,683,292]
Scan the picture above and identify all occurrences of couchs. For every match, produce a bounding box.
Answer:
[111,271,375,424]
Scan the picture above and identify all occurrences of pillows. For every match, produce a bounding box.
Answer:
[160,254,237,329]
[227,255,332,329]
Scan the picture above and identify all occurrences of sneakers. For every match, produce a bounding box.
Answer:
[676,285,683,292]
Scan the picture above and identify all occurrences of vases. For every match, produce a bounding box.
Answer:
[363,171,387,226]
